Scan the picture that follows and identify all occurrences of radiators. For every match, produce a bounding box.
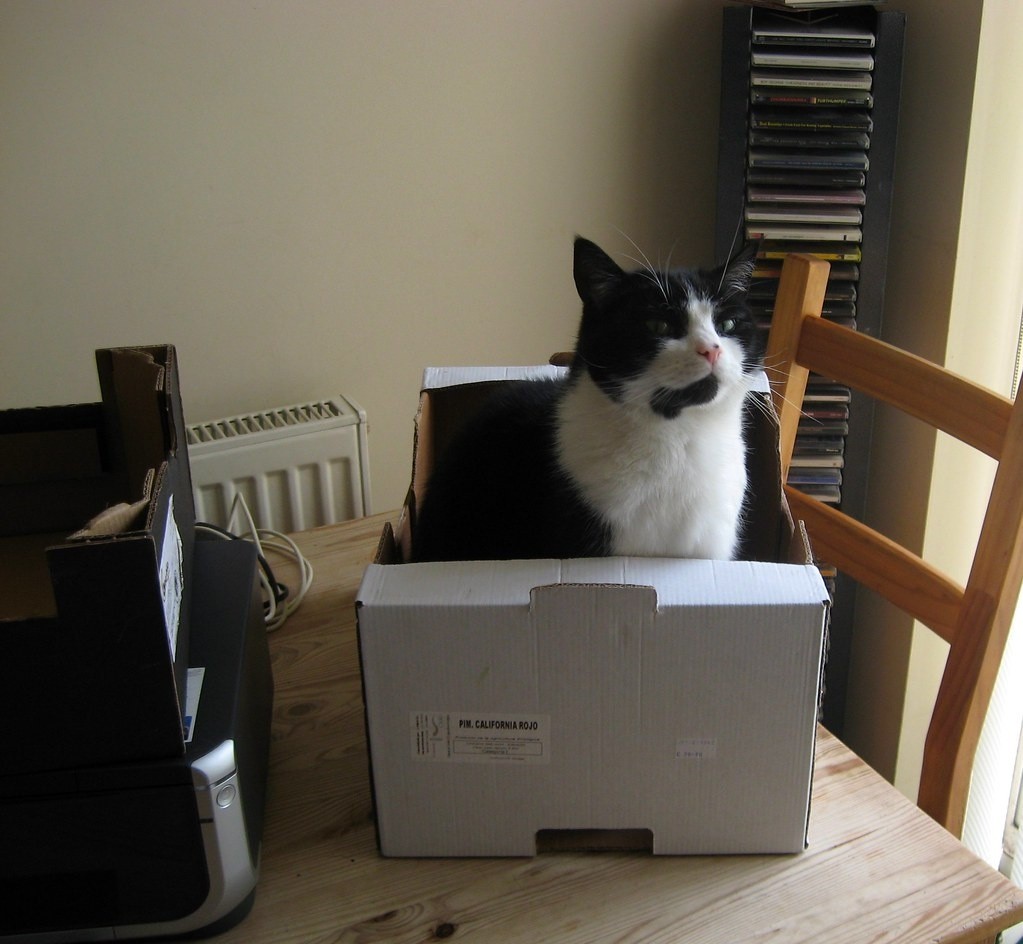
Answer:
[185,396,372,539]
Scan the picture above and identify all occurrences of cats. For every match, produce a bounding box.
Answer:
[411,232,768,560]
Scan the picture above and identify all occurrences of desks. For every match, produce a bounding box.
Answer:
[223,506,1023,944]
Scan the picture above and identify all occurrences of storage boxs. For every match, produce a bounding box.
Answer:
[352,364,833,859]
[0,342,199,775]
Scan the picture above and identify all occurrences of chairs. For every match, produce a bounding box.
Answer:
[758,252,1023,842]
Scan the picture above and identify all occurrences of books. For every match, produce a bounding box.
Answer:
[740,0,878,724]
